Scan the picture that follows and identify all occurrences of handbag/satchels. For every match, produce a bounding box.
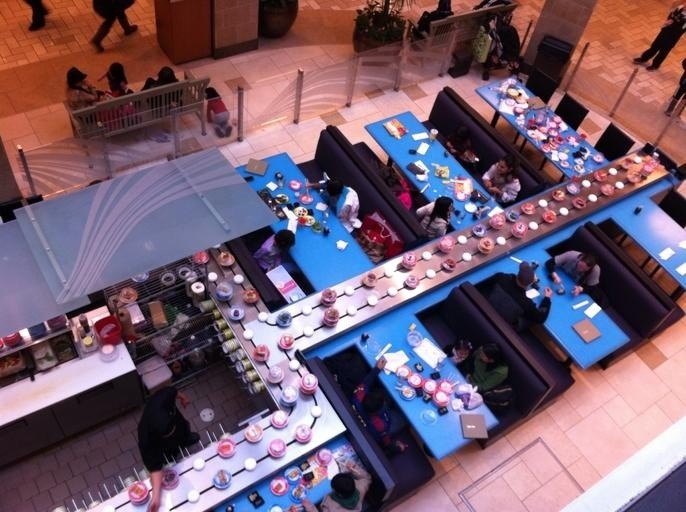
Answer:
[356,209,403,263]
[470,12,520,63]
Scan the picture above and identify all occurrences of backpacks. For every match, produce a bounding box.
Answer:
[484,387,513,418]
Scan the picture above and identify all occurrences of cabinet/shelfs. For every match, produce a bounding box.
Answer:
[0,371,145,467]
[102,256,224,391]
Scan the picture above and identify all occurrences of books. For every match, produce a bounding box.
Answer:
[525,97,546,111]
[571,318,603,344]
[244,158,269,176]
[459,412,489,440]
[382,119,408,137]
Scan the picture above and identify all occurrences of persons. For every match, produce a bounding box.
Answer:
[412,195,455,240]
[65,60,235,142]
[378,162,413,211]
[253,228,303,281]
[442,337,475,382]
[632,1,686,71]
[409,0,526,80]
[480,264,553,336]
[544,250,601,297]
[298,458,373,511]
[465,336,511,394]
[137,384,201,512]
[480,153,522,208]
[22,0,50,32]
[304,176,361,230]
[90,0,139,54]
[352,355,411,459]
[662,57,686,123]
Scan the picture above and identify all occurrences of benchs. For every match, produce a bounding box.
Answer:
[63,70,211,168]
[296,125,432,265]
[421,86,553,209]
[420,281,575,451]
[226,236,312,312]
[404,0,519,65]
[307,355,436,511]
[552,221,677,370]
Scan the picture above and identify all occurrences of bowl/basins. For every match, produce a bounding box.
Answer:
[344,154,654,317]
[501,86,597,153]
[191,249,339,405]
[124,409,316,512]
[396,363,484,404]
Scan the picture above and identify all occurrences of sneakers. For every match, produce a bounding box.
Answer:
[29,11,47,31]
[89,33,104,52]
[633,58,659,71]
[122,23,137,35]
[215,126,233,137]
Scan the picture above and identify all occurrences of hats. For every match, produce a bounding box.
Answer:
[518,262,534,286]
[67,67,87,84]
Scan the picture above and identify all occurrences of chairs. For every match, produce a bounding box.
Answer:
[525,66,558,105]
[657,188,686,228]
[554,93,588,131]
[594,123,636,160]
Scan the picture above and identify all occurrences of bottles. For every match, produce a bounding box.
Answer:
[79,313,90,334]
